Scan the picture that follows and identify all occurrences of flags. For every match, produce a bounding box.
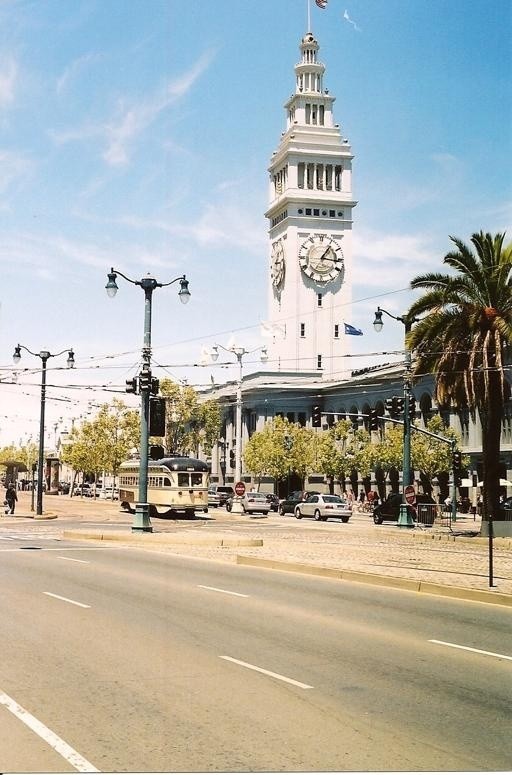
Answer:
[315,0,328,8]
[344,322,364,337]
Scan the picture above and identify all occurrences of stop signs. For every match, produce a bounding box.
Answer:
[404,485,416,505]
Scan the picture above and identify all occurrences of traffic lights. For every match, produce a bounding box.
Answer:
[408,397,415,415]
[396,398,404,412]
[140,369,151,392]
[384,398,393,410]
[454,472,460,485]
[284,435,292,449]
[452,453,460,470]
[311,405,321,427]
[124,376,137,395]
[368,408,378,431]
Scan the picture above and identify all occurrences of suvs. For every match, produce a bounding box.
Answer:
[209,485,235,507]
[373,494,437,527]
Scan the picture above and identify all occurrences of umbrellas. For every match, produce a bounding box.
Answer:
[478,478,512,489]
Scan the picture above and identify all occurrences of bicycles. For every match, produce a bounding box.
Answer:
[358,499,371,513]
[363,500,374,513]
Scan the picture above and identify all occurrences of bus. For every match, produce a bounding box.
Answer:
[116,453,208,517]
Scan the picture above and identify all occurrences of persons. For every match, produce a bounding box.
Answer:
[5,482,19,515]
[334,487,511,512]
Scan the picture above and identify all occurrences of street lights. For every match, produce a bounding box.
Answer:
[235,483,245,496]
[372,306,434,527]
[106,267,191,533]
[211,342,268,483]
[13,342,77,516]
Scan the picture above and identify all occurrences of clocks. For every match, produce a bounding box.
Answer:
[269,242,286,289]
[298,234,345,283]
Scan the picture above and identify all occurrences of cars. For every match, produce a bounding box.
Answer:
[294,495,352,523]
[19,479,118,500]
[500,497,511,510]
[264,493,279,512]
[208,490,220,509]
[226,492,271,515]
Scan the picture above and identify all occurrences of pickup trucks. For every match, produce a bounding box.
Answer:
[279,490,320,515]
